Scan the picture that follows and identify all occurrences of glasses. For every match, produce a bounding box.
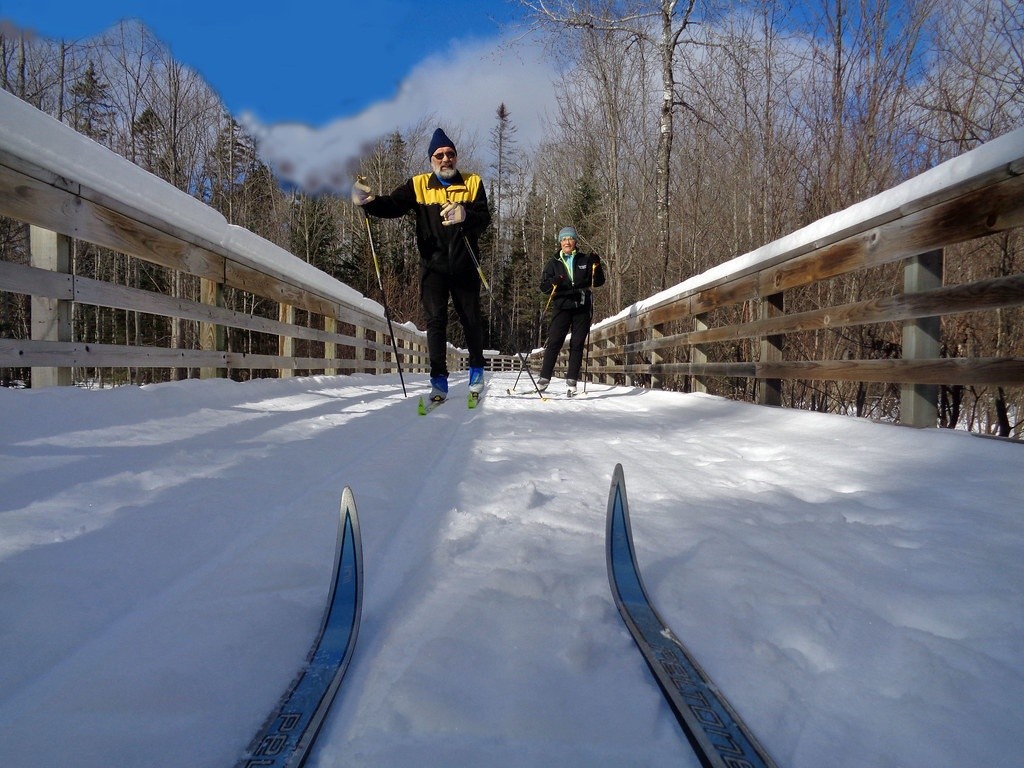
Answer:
[560,238,575,242]
[432,151,456,160]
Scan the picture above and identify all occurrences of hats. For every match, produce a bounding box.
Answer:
[558,226,577,243]
[428,128,457,162]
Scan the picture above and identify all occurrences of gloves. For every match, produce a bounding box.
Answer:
[590,251,600,263]
[549,276,561,285]
[351,176,375,206]
[439,202,466,225]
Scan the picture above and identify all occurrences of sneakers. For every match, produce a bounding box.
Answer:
[533,377,550,392]
[468,364,484,393]
[566,378,577,392]
[429,373,448,400]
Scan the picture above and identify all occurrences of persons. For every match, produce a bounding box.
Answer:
[352,128,491,402]
[536,226,606,396]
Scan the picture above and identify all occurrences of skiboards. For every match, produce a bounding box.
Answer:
[233,462,780,768]
[506,377,578,401]
[418,367,485,415]
